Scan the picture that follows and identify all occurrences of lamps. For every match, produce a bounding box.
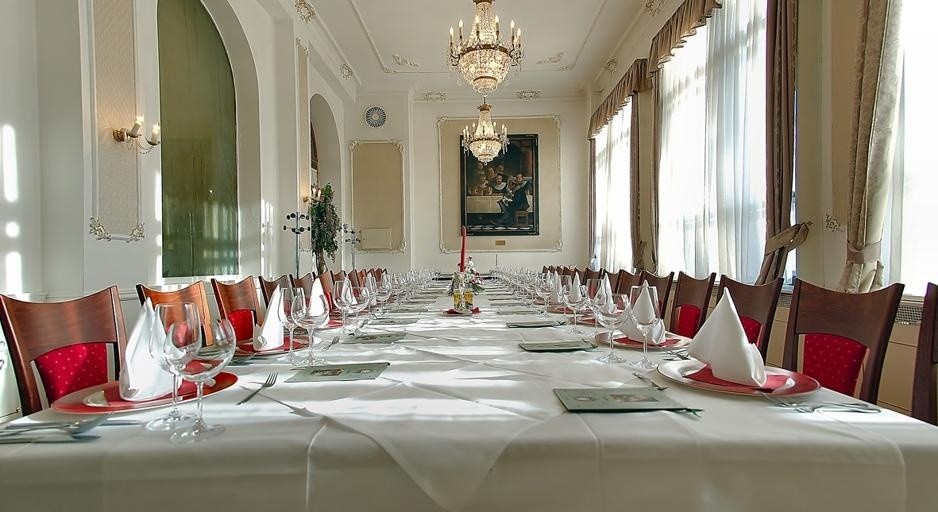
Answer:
[113,114,161,154]
[462,98,511,165]
[446,0,528,97]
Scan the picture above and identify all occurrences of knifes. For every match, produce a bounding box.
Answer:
[5,420,141,428]
[0,434,102,445]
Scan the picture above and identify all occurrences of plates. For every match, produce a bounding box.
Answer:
[534,300,551,305]
[570,315,602,325]
[547,306,573,313]
[51,372,238,415]
[656,358,819,397]
[595,332,686,350]
[325,321,342,329]
[238,335,313,355]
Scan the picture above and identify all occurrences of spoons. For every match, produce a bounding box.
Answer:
[0,412,113,437]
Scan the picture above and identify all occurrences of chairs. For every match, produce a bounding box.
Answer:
[135,282,211,345]
[782,277,905,405]
[717,274,783,364]
[291,268,392,312]
[670,272,716,337]
[911,282,938,427]
[259,275,295,314]
[0,286,128,421]
[540,264,674,320]
[221,275,261,338]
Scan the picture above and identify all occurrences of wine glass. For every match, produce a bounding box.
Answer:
[629,286,659,369]
[562,285,588,335]
[555,275,572,315]
[489,265,535,304]
[277,288,306,362]
[382,274,392,309]
[592,293,629,362]
[291,295,329,366]
[163,320,237,446]
[333,281,352,335]
[361,277,377,318]
[344,287,369,336]
[534,279,553,317]
[585,279,606,337]
[373,276,392,317]
[147,303,201,434]
[392,267,440,305]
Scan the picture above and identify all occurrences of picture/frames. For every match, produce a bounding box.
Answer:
[460,133,540,236]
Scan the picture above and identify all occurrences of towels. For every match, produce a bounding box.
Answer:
[686,286,766,386]
[250,284,284,350]
[120,295,187,400]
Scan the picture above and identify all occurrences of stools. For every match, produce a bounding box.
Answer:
[516,212,528,225]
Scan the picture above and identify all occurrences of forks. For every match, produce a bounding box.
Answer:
[237,373,278,406]
[795,402,869,413]
[762,392,881,415]
[324,336,341,351]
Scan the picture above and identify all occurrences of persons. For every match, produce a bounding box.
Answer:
[467,161,532,227]
